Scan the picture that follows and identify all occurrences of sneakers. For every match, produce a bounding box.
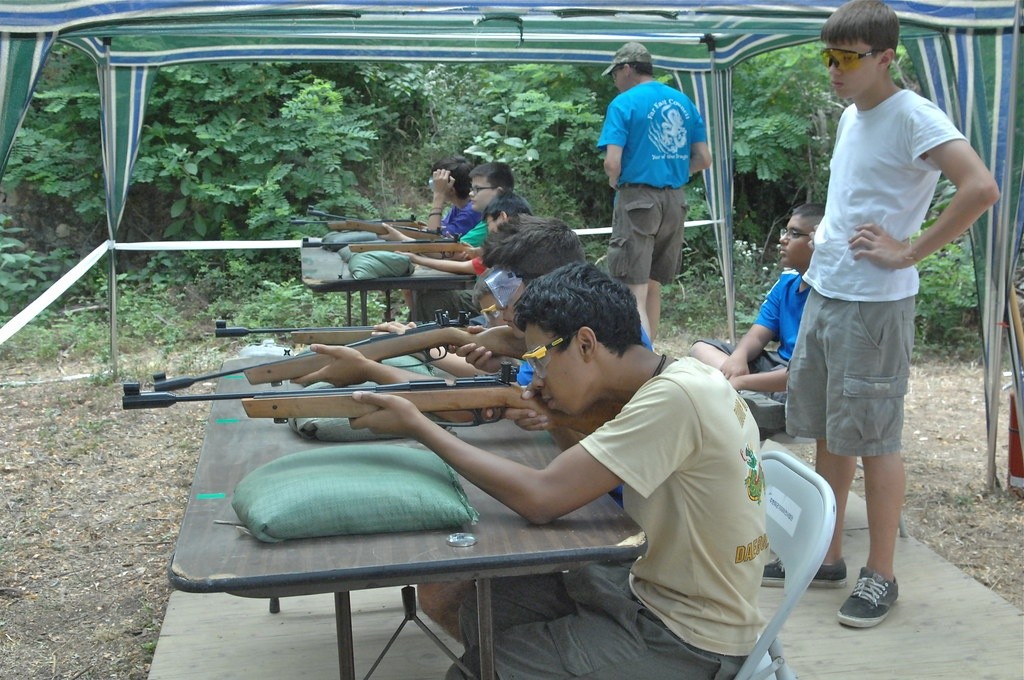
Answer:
[763,557,847,588]
[837,567,899,628]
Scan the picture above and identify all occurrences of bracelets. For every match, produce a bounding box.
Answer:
[905,240,918,263]
[429,212,443,217]
[430,206,443,211]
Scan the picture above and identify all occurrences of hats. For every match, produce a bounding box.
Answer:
[601,42,656,76]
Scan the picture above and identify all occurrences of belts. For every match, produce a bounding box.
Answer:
[618,183,665,190]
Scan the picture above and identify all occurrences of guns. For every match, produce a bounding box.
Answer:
[301,233,486,259]
[214,307,474,348]
[120,360,632,435]
[306,204,447,240]
[290,214,429,232]
[152,308,533,395]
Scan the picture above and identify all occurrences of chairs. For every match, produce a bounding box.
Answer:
[733,450,838,680]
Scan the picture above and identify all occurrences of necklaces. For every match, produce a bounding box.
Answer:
[652,353,667,376]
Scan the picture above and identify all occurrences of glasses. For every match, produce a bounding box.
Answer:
[522,330,576,380]
[485,268,522,306]
[470,184,495,194]
[610,66,625,76]
[821,47,881,70]
[778,227,809,242]
[478,305,501,324]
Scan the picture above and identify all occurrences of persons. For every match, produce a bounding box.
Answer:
[762,0,1001,630]
[290,160,770,680]
[597,42,711,344]
[688,202,826,442]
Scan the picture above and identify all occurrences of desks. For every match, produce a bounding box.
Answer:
[299,248,477,325]
[168,354,647,680]
